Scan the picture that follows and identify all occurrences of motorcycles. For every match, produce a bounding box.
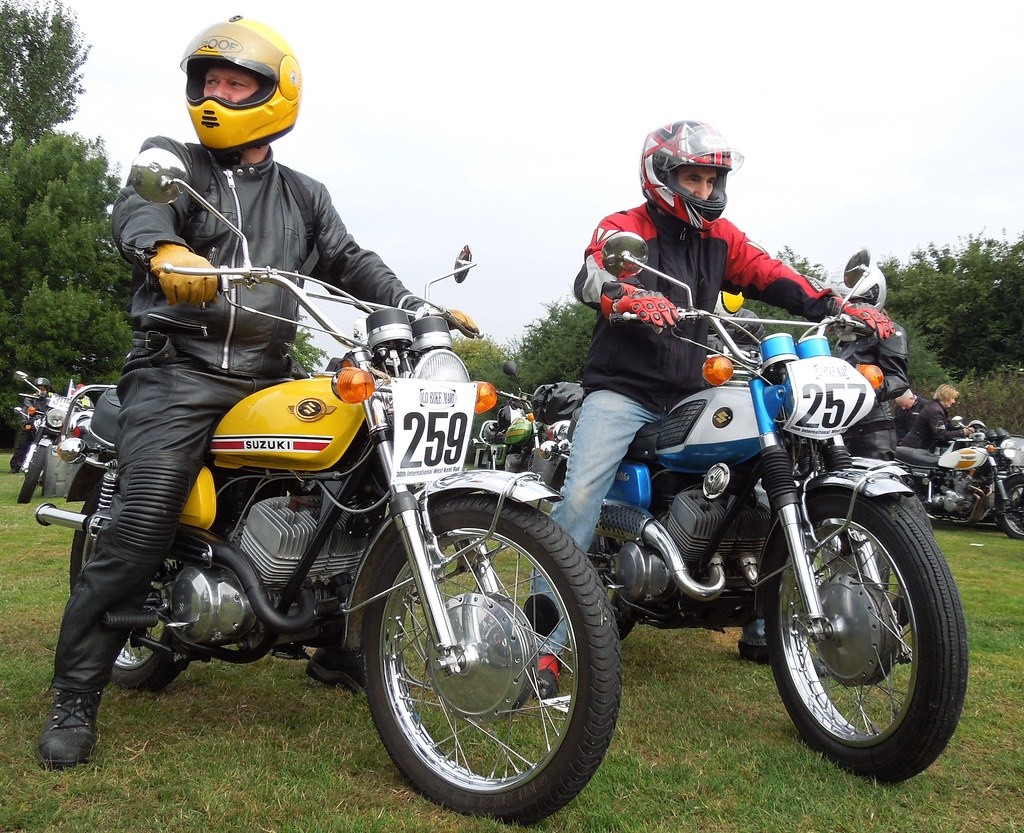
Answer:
[465,359,583,475]
[520,229,969,780]
[889,413,1024,541]
[12,369,97,504]
[36,146,629,825]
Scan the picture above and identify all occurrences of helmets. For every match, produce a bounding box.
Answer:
[77,384,86,397]
[178,15,300,149]
[638,121,732,231]
[840,268,887,312]
[34,378,50,390]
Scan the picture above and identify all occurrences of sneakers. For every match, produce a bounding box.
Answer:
[306,644,368,697]
[38,691,102,768]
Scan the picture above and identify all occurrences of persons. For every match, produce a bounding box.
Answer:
[894,384,975,454]
[522,121,894,699]
[36,14,483,769]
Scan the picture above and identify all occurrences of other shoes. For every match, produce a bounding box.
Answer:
[7,468,19,473]
[738,639,829,678]
[522,593,562,674]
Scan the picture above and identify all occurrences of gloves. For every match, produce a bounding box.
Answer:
[148,244,219,307]
[600,277,682,328]
[825,296,895,340]
[449,309,479,335]
